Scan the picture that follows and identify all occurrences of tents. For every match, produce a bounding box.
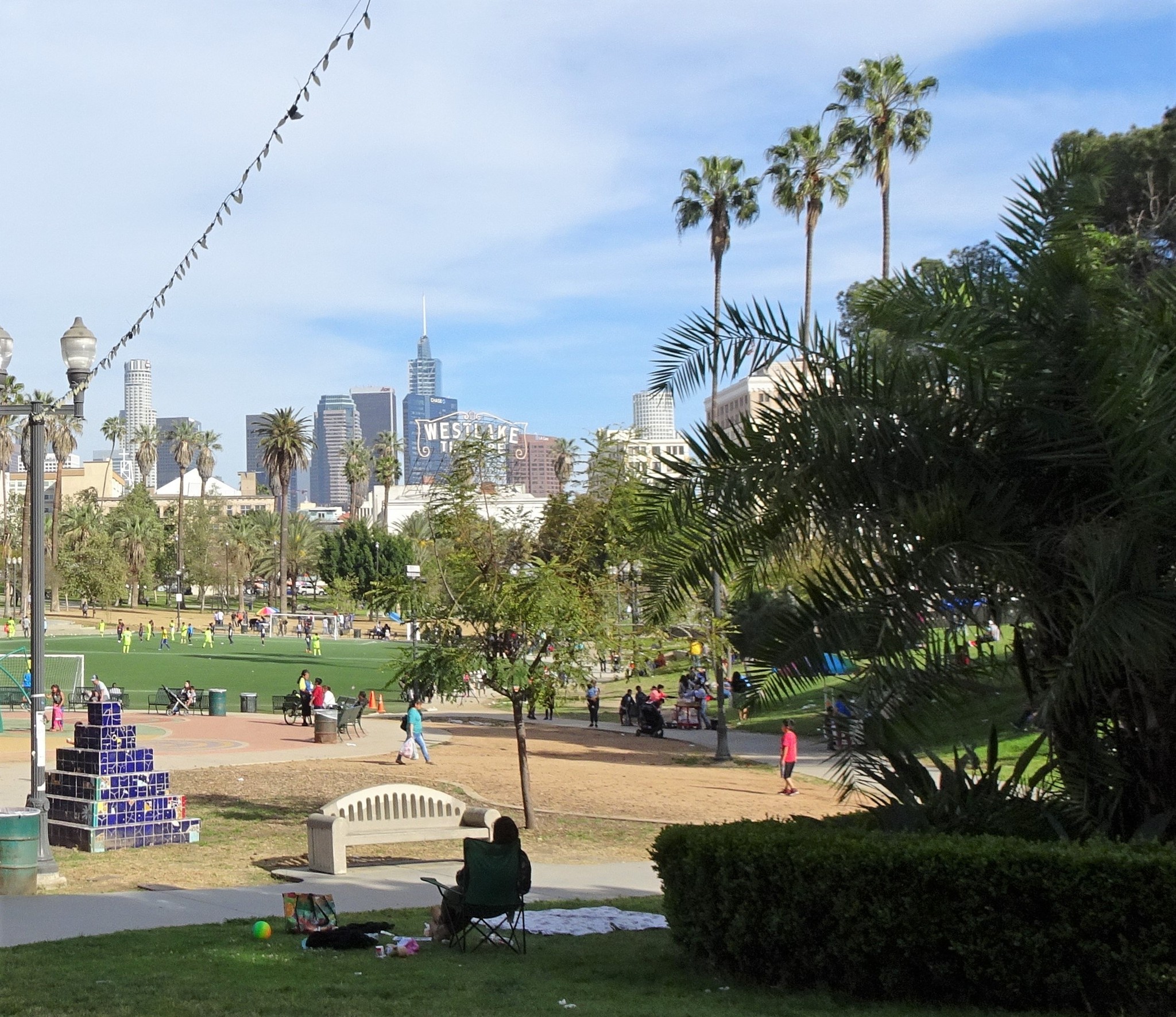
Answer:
[778,651,861,675]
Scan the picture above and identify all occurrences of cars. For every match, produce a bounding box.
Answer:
[285,585,299,595]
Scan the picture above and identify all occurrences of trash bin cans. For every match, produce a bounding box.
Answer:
[240,692,257,712]
[208,688,227,716]
[0,806,41,896]
[353,628,361,638]
[313,708,339,744]
[241,624,248,634]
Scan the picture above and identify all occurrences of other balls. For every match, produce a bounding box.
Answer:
[251,921,272,941]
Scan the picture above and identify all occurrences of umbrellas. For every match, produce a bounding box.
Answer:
[256,607,280,621]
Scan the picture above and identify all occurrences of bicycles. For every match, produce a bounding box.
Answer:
[283,699,313,725]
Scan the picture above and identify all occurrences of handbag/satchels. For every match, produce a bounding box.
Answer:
[399,737,419,760]
[400,715,408,731]
[282,892,338,933]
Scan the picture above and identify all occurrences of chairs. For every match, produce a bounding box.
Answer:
[421,876,528,956]
[334,696,366,742]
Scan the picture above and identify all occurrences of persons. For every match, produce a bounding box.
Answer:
[598,640,666,677]
[396,698,435,764]
[22,653,32,703]
[5,615,47,638]
[45,684,64,733]
[730,671,748,725]
[585,679,600,728]
[297,669,369,734]
[181,681,196,708]
[459,626,576,720]
[81,596,390,655]
[430,816,532,942]
[778,718,799,796]
[969,620,1000,647]
[90,675,122,705]
[619,684,669,726]
[824,694,852,751]
[678,638,735,730]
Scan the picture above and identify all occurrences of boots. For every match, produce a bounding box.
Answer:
[429,921,451,942]
[431,906,444,925]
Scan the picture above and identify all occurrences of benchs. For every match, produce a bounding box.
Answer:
[306,782,501,875]
[147,688,209,715]
[363,629,397,641]
[0,686,31,712]
[68,687,129,712]
[271,695,302,714]
[291,626,317,634]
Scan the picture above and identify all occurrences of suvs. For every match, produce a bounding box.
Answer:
[298,585,328,595]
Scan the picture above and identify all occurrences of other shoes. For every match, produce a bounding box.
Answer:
[704,726,711,729]
[396,759,405,765]
[696,727,701,729]
[788,788,799,796]
[426,761,435,765]
[589,724,593,726]
[543,717,548,720]
[549,718,552,720]
[595,725,597,726]
[531,716,536,719]
[778,788,791,795]
[527,715,532,718]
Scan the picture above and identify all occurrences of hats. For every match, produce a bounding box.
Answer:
[91,675,98,681]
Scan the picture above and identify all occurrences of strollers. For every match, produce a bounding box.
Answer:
[161,684,191,716]
[635,704,666,739]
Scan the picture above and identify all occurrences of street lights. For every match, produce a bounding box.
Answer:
[175,570,183,632]
[374,541,381,621]
[5,556,23,621]
[704,498,734,761]
[274,541,278,608]
[406,564,422,664]
[225,542,230,613]
[0,315,99,877]
[175,537,179,608]
